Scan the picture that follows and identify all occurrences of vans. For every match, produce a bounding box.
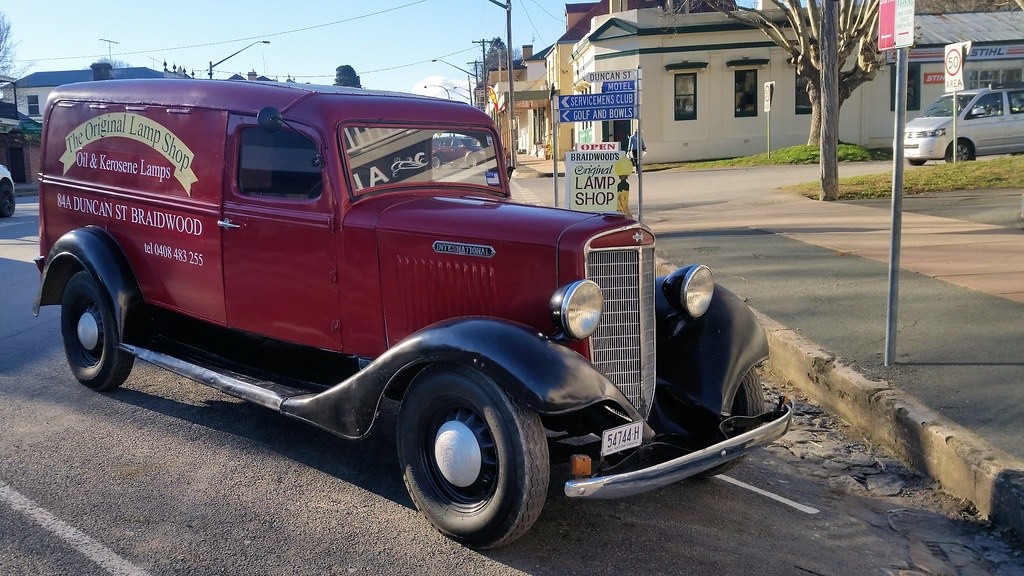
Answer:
[902,82,1024,167]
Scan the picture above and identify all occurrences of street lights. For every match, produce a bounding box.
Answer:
[432,59,479,88]
[453,86,472,105]
[424,85,451,99]
[209,41,271,79]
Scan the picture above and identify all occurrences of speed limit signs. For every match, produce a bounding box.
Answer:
[763,82,771,113]
[943,41,964,93]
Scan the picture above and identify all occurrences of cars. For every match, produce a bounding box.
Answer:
[31,78,793,553]
[431,137,486,168]
[0,165,16,218]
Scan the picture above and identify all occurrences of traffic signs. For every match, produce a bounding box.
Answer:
[583,68,637,83]
[877,0,916,51]
[601,80,636,93]
[558,92,635,110]
[559,105,637,123]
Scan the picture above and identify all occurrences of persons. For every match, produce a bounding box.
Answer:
[629,129,647,173]
[959,100,972,115]
